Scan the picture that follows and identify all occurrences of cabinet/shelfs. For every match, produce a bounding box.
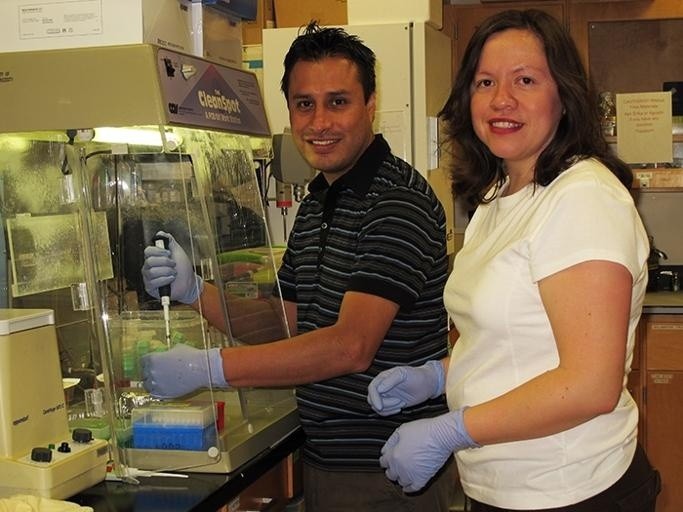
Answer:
[441,1,682,191]
[626,307,682,512]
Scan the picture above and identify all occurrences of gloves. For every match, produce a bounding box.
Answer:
[140,229,205,306]
[378,403,484,496]
[365,358,445,418]
[136,341,232,401]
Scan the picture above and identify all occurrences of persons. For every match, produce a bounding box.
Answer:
[367,9,662,512]
[139,27,457,512]
